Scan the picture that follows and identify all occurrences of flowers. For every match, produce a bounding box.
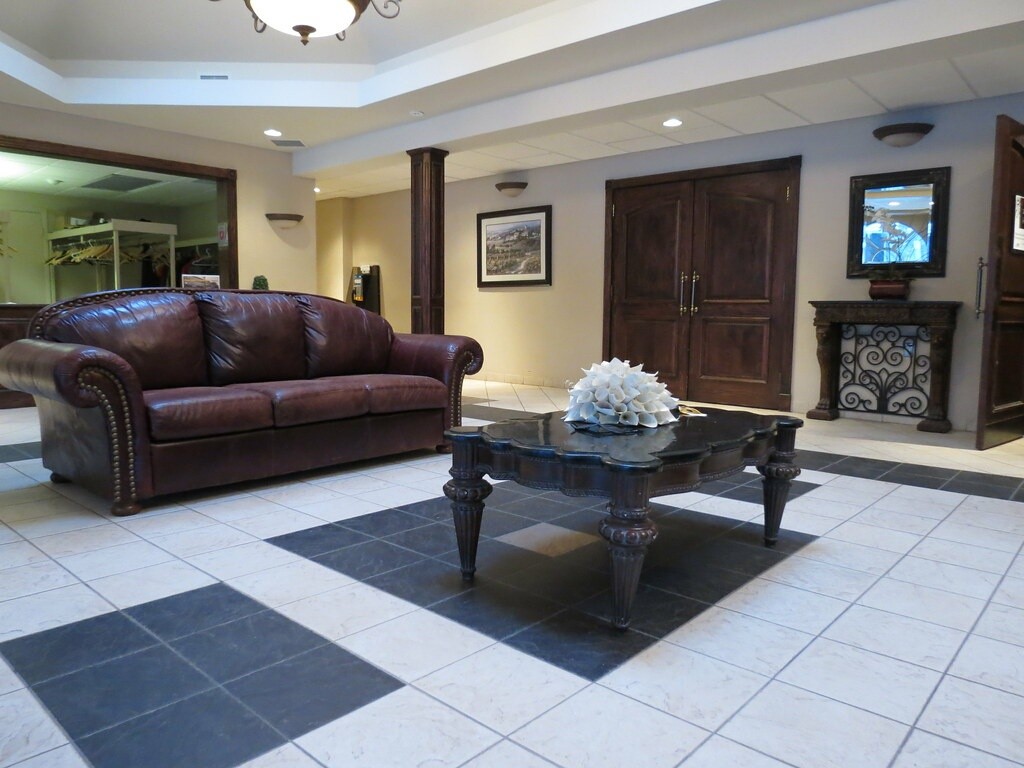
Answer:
[561,359,681,429]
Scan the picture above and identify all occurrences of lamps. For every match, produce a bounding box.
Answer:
[266,214,304,229]
[244,0,400,47]
[496,181,528,197]
[871,122,935,146]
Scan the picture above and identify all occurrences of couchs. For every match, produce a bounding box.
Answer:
[0,287,483,515]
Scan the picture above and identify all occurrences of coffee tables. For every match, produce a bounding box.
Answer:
[443,404,803,630]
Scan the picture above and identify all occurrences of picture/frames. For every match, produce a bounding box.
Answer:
[846,165,949,279]
[476,205,552,288]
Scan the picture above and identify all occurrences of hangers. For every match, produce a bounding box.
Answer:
[43,241,170,266]
[194,249,219,268]
